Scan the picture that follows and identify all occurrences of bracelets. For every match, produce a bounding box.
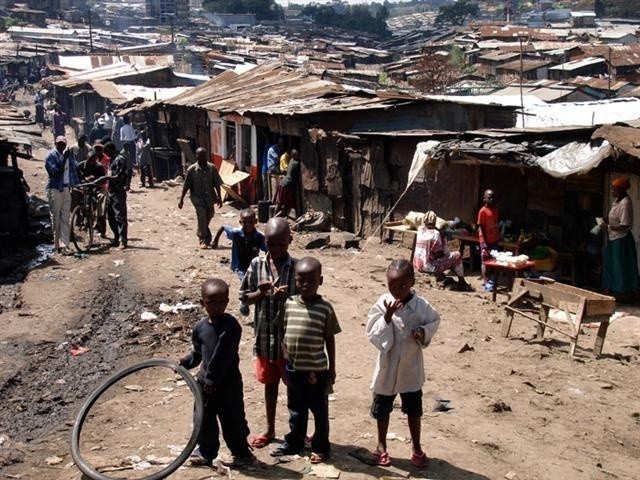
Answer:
[478,231,488,250]
[607,224,612,232]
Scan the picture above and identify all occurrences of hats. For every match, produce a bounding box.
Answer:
[56,136,67,145]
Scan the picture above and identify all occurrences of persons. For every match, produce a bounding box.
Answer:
[478,189,499,291]
[34,90,136,256]
[598,175,640,299]
[365,259,441,467]
[22,109,33,158]
[177,146,223,249]
[261,137,303,219]
[414,210,476,292]
[238,217,313,448]
[136,129,153,188]
[212,208,267,315]
[269,257,342,464]
[173,278,267,467]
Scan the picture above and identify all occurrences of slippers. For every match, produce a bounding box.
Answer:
[185,430,429,467]
[240,302,248,315]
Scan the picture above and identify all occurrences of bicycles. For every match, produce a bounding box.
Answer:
[69,184,103,253]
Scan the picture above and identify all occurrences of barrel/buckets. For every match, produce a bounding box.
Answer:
[269,205,276,218]
[258,200,271,222]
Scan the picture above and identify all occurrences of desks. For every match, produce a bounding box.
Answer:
[453,235,520,272]
[385,225,418,261]
[483,259,535,302]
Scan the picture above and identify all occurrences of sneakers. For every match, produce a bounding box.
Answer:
[60,246,72,256]
[485,283,494,292]
[111,240,119,246]
[458,280,471,287]
[102,231,104,237]
[199,240,208,248]
[487,280,494,283]
[119,243,125,251]
